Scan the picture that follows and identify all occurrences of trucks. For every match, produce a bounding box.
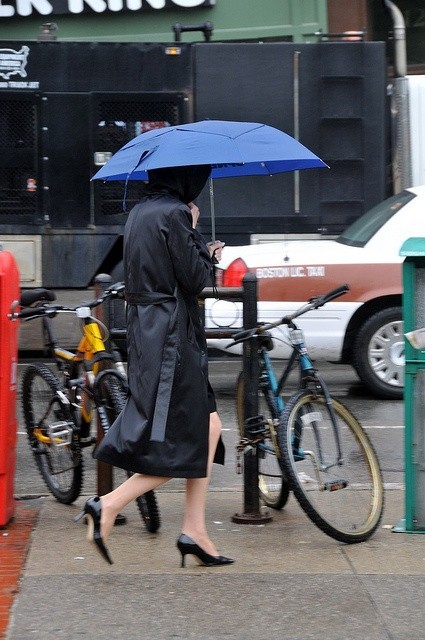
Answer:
[0,23,425,349]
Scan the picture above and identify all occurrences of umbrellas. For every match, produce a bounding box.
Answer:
[88,121,332,266]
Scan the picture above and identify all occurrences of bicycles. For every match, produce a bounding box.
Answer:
[5,278,163,534]
[224,283,384,544]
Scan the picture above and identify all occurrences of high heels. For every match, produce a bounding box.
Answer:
[72,495,115,566]
[176,534,236,568]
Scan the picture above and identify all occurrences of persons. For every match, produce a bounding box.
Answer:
[74,164,236,567]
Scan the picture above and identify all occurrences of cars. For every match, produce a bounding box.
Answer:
[204,184,425,402]
